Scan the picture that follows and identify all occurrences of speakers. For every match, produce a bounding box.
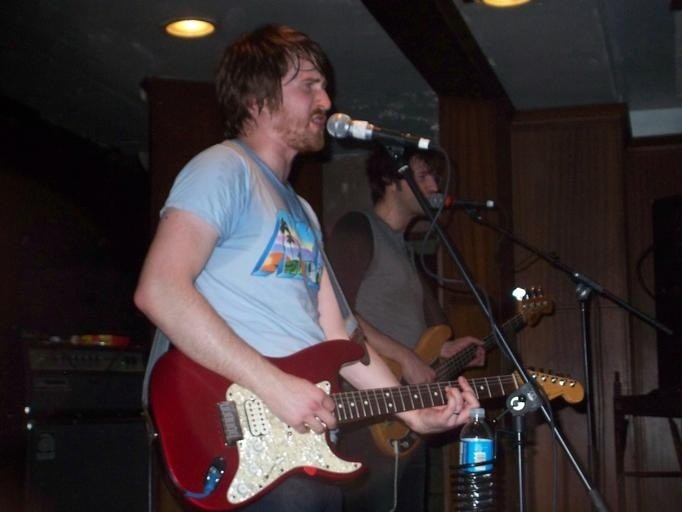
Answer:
[22,413,155,511]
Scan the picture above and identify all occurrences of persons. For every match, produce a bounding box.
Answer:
[134,19,482,511]
[325,138,487,511]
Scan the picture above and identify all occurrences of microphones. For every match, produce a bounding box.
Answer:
[327,113,429,152]
[429,193,494,210]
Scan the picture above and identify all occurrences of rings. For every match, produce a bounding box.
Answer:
[452,412,460,415]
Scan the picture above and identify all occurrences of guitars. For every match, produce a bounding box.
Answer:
[369,286,555,458]
[148,339,584,512]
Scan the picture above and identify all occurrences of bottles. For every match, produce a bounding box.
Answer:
[454,405,498,512]
